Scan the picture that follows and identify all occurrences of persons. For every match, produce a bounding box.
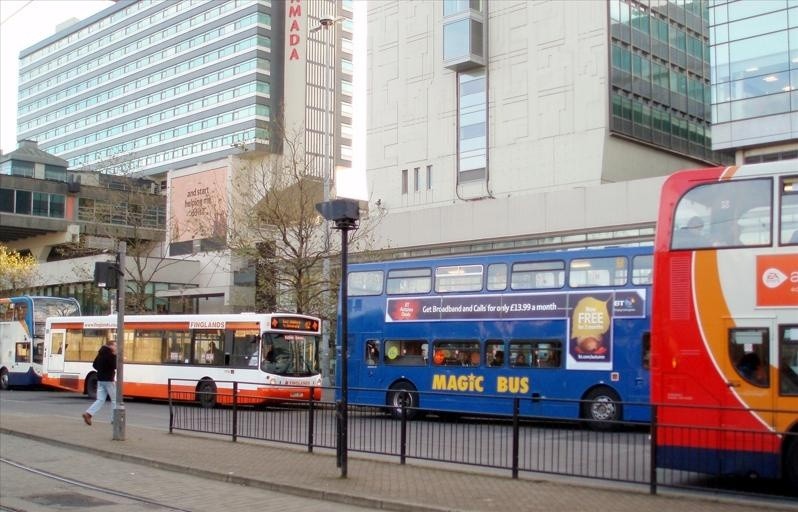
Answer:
[673,216,713,248]
[82,340,117,426]
[736,352,768,386]
[205,342,220,364]
[715,223,745,246]
[490,351,504,366]
[513,353,530,368]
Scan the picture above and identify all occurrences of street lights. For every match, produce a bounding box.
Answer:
[309,15,345,386]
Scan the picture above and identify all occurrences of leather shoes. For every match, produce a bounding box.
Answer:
[82,412,92,425]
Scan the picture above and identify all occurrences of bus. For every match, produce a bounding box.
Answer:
[335,247,654,431]
[40,311,323,410]
[0,295,81,390]
[650,159,798,498]
